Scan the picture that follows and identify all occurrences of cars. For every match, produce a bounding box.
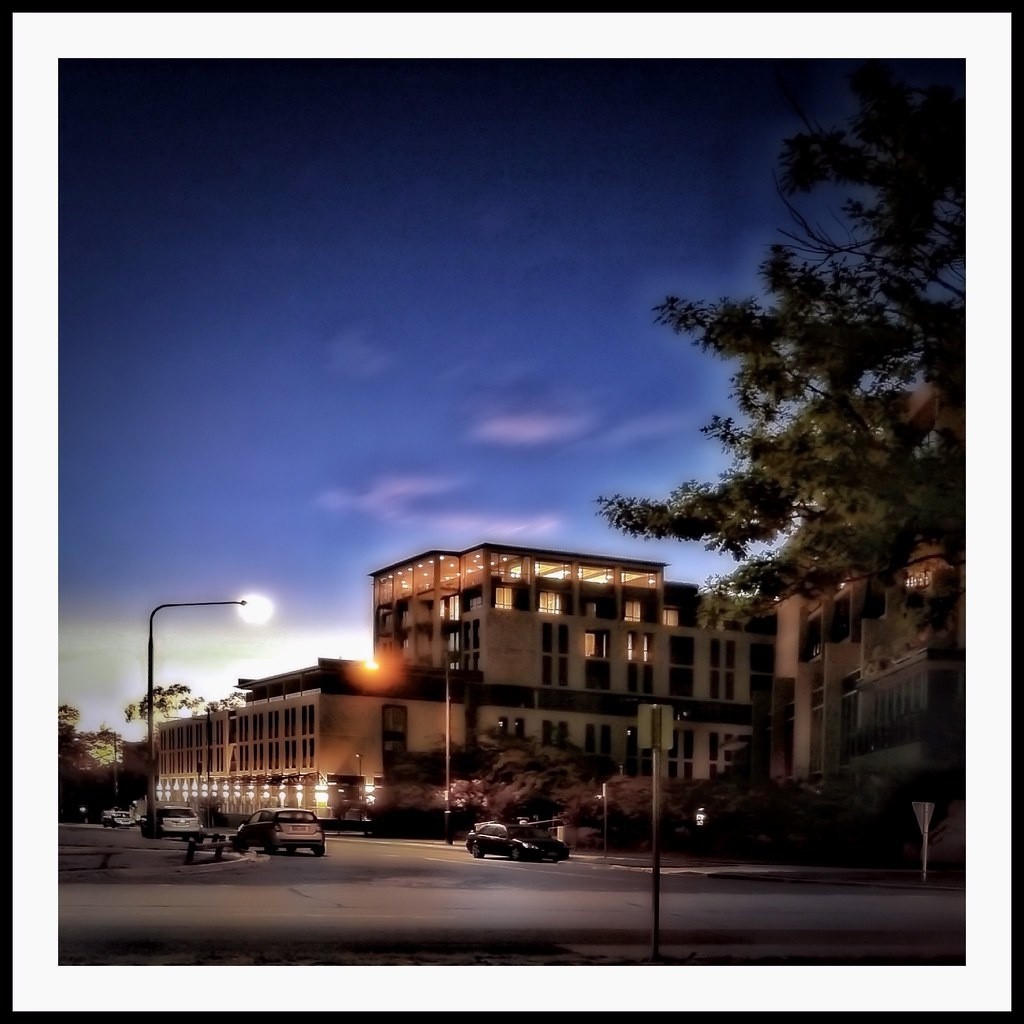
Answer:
[238,807,325,857]
[142,805,204,844]
[103,810,135,828]
[465,823,570,862]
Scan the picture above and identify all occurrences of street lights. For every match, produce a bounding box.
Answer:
[356,753,364,774]
[148,600,247,840]
